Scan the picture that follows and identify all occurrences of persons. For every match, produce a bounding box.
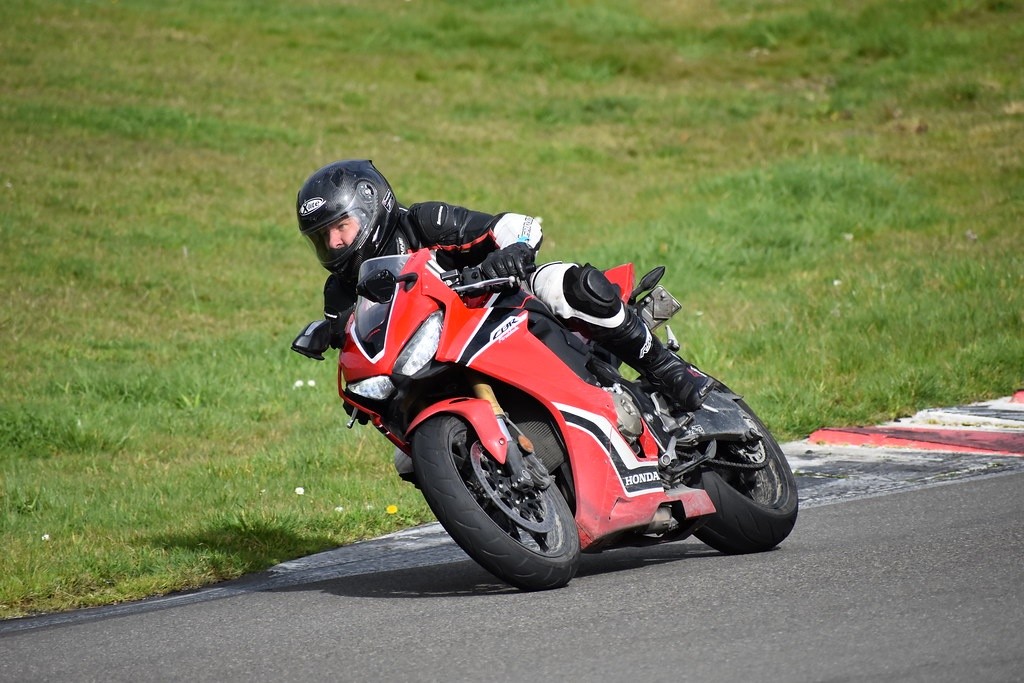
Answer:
[296,159,715,488]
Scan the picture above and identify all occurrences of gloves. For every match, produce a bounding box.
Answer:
[480,242,535,292]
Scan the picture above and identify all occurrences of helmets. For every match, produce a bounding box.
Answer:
[296,159,401,284]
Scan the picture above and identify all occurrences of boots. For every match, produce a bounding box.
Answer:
[597,307,714,410]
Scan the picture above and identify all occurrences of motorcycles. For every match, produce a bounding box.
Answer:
[287,245,799,593]
